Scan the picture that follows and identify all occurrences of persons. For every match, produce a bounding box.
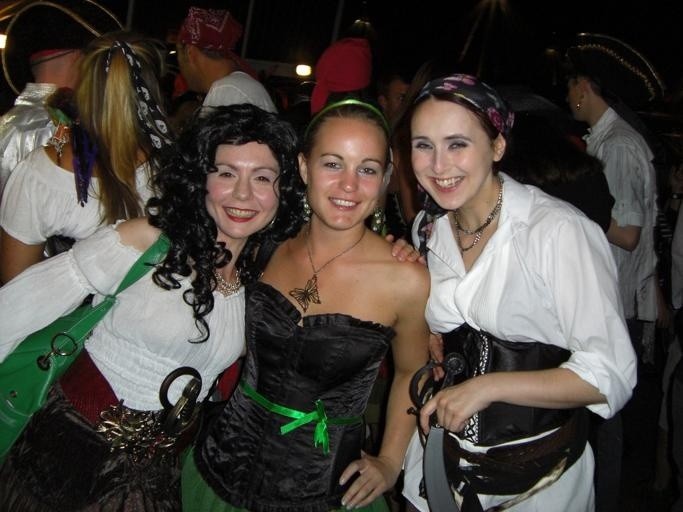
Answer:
[1,5,681,512]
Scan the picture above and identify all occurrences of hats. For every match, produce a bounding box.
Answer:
[2,2,125,94]
[564,31,664,103]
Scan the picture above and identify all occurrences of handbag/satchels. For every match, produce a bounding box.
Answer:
[0,302,113,460]
[178,398,225,443]
